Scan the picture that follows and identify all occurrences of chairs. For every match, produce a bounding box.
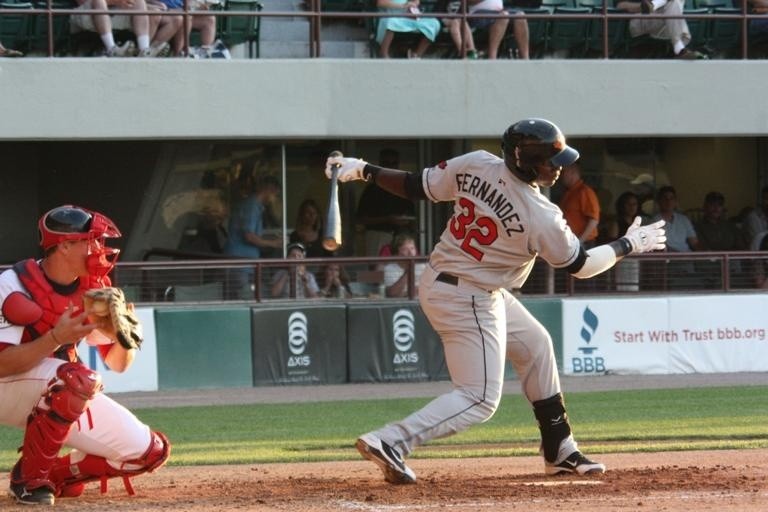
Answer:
[0,0,766,60]
[105,218,414,308]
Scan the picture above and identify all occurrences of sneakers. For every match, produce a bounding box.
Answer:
[199,48,223,59]
[109,39,136,58]
[138,42,171,58]
[8,481,54,507]
[355,431,416,485]
[543,448,605,478]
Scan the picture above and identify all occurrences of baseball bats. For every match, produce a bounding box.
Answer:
[321,151,343,252]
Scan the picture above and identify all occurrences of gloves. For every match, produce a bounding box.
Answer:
[624,215,668,253]
[325,155,368,183]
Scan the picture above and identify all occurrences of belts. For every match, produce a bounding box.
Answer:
[435,273,459,285]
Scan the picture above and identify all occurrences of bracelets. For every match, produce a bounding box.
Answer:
[45,329,63,350]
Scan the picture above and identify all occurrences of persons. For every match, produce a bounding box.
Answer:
[65,0,229,60]
[0,204,173,506]
[372,1,442,60]
[550,161,767,295]
[430,1,481,61]
[611,0,710,62]
[465,1,531,61]
[320,118,670,485]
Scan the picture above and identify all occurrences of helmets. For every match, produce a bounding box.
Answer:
[500,118,581,183]
[35,204,122,283]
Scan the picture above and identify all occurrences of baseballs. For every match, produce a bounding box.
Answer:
[93,300,109,317]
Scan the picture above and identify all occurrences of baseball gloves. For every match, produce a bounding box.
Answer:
[84,286,143,349]
